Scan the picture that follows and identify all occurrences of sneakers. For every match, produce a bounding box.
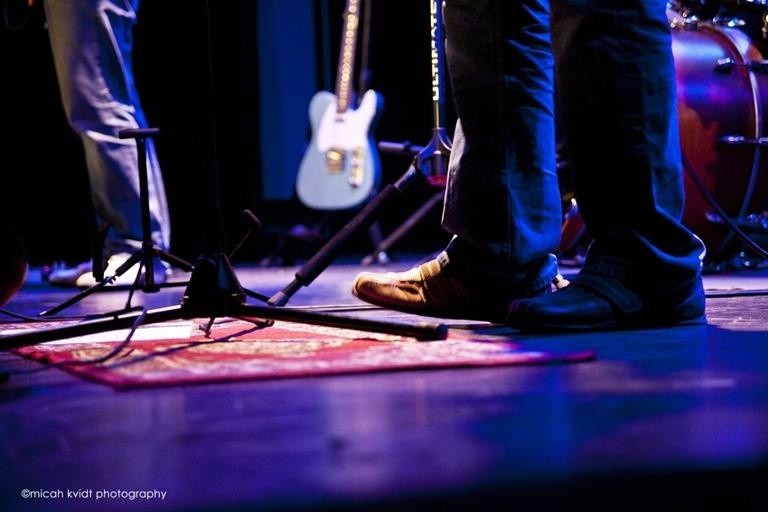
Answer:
[351,258,563,321]
[49,249,167,286]
[507,247,707,334]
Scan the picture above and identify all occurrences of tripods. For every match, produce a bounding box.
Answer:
[267,2,455,304]
[1,0,448,382]
[36,126,270,313]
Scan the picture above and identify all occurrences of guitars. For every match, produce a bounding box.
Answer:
[296,0,384,210]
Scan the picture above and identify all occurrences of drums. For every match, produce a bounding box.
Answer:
[673,16,768,265]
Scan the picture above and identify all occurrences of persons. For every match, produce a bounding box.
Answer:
[42,0,171,289]
[351,0,704,335]
